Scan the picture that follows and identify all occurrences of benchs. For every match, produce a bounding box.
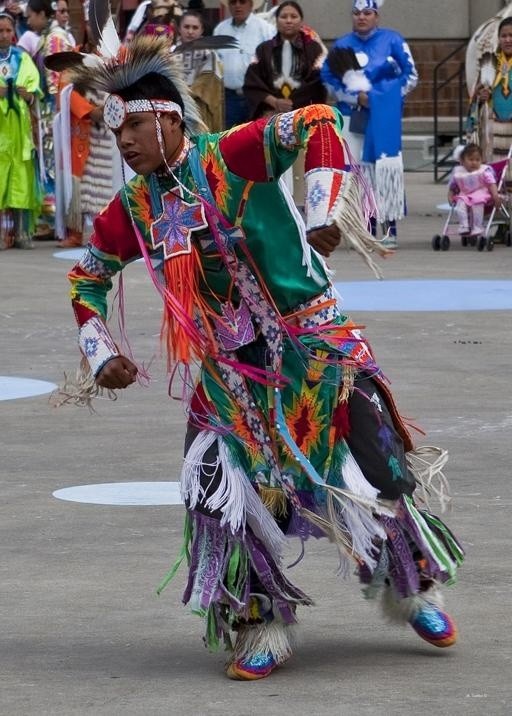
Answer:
[401,134,435,173]
[401,115,467,147]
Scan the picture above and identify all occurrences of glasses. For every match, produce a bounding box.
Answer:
[56,8,70,14]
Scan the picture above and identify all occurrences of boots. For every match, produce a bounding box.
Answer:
[0,212,34,250]
[57,216,84,247]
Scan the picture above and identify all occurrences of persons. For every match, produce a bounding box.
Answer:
[2,1,329,252]
[319,0,419,258]
[467,16,512,182]
[448,143,500,235]
[67,64,466,681]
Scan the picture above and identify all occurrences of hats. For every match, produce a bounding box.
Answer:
[110,71,185,126]
[351,0,378,14]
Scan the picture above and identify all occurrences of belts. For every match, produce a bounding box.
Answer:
[225,87,244,96]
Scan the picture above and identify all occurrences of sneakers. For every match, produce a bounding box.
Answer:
[227,648,292,681]
[406,603,456,648]
[458,225,482,236]
[377,236,398,248]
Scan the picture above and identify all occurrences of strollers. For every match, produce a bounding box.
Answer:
[432,143,511,253]
